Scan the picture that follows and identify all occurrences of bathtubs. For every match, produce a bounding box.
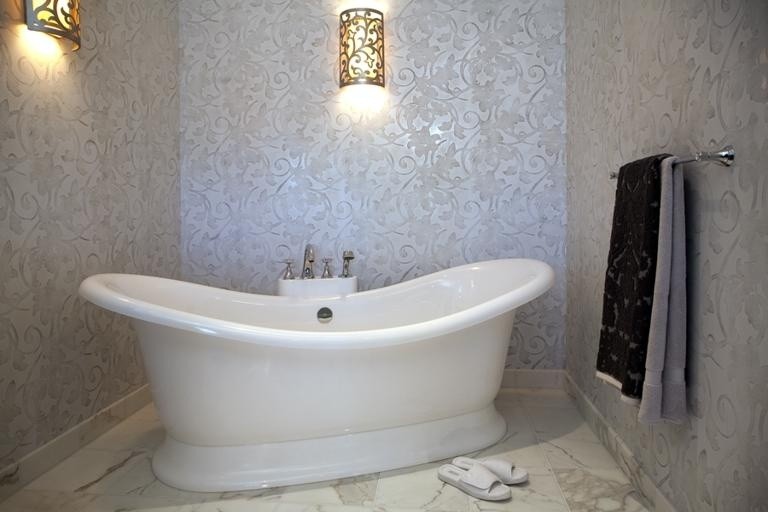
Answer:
[78,257,556,494]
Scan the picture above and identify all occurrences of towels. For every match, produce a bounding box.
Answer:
[591,153,695,427]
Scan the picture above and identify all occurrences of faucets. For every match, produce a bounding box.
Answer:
[302,244,314,277]
[343,249,355,277]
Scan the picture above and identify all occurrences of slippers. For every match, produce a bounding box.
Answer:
[437,463,511,501]
[452,456,528,484]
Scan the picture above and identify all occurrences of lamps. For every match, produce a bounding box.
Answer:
[21,0,83,57]
[331,7,388,89]
